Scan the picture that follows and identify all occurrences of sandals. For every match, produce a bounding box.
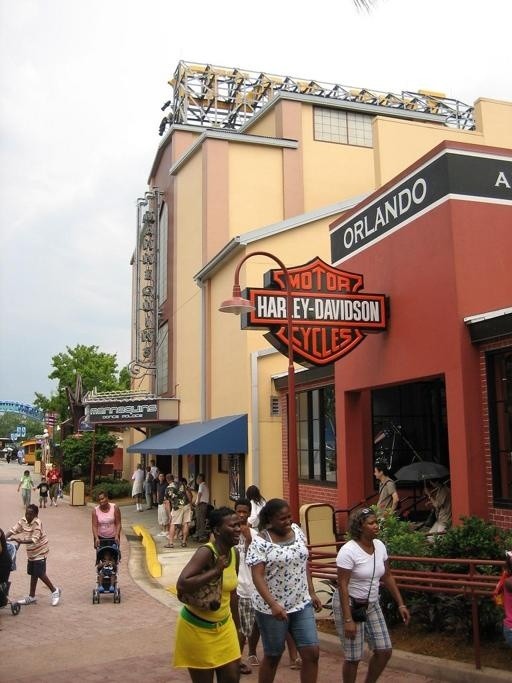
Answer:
[164,543,174,548]
[290,657,303,669]
[240,654,260,674]
[181,541,187,547]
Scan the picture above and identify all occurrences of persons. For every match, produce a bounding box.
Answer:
[97,551,117,593]
[17,447,24,465]
[374,462,399,514]
[332,506,410,683]
[47,464,64,507]
[35,478,50,509]
[6,451,11,464]
[235,485,268,676]
[6,503,61,606]
[17,470,35,508]
[91,491,122,550]
[424,479,452,541]
[245,499,323,682]
[172,508,242,683]
[132,458,209,548]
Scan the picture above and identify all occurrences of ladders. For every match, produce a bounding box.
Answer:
[389,421,423,470]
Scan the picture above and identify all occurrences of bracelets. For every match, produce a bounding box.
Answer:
[397,606,406,609]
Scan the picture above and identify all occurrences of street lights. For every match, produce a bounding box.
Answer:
[42,427,50,463]
[73,415,94,503]
[217,250,301,526]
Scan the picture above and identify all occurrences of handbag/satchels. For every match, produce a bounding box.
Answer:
[349,595,369,622]
[177,571,222,611]
[58,488,63,498]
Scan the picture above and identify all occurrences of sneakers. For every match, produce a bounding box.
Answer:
[134,501,158,512]
[51,586,62,606]
[39,499,58,508]
[17,595,37,605]
[157,530,183,539]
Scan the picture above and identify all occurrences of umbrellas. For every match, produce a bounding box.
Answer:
[394,462,450,489]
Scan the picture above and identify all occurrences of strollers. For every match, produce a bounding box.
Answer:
[94,538,122,604]
[0,530,22,615]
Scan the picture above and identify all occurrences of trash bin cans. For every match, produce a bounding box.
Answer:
[299,502,337,568]
[34,461,40,474]
[2,450,13,459]
[70,479,85,506]
